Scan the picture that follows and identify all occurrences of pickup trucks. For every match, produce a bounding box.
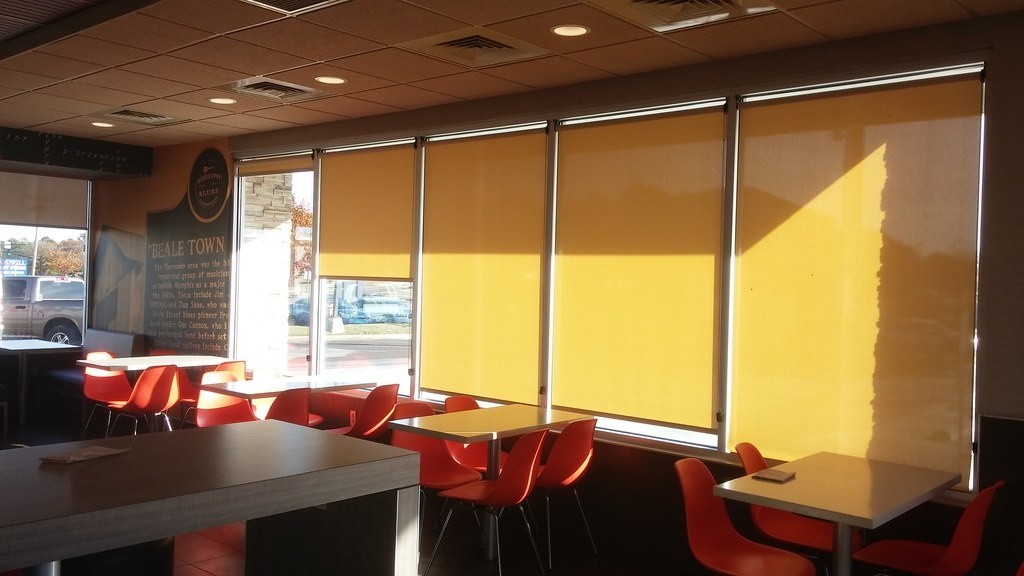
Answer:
[0,275,84,346]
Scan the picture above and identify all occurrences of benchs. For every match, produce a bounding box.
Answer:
[30,328,145,429]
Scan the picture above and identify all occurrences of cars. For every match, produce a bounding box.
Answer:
[288,295,372,327]
[347,297,413,323]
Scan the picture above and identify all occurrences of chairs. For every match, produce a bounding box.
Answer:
[82,348,600,576]
[852,479,1005,576]
[675,458,816,576]
[734,443,863,574]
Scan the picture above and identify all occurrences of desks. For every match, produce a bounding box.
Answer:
[386,404,593,561]
[0,420,423,576]
[0,338,82,428]
[197,373,376,400]
[75,353,236,434]
[712,451,961,576]
